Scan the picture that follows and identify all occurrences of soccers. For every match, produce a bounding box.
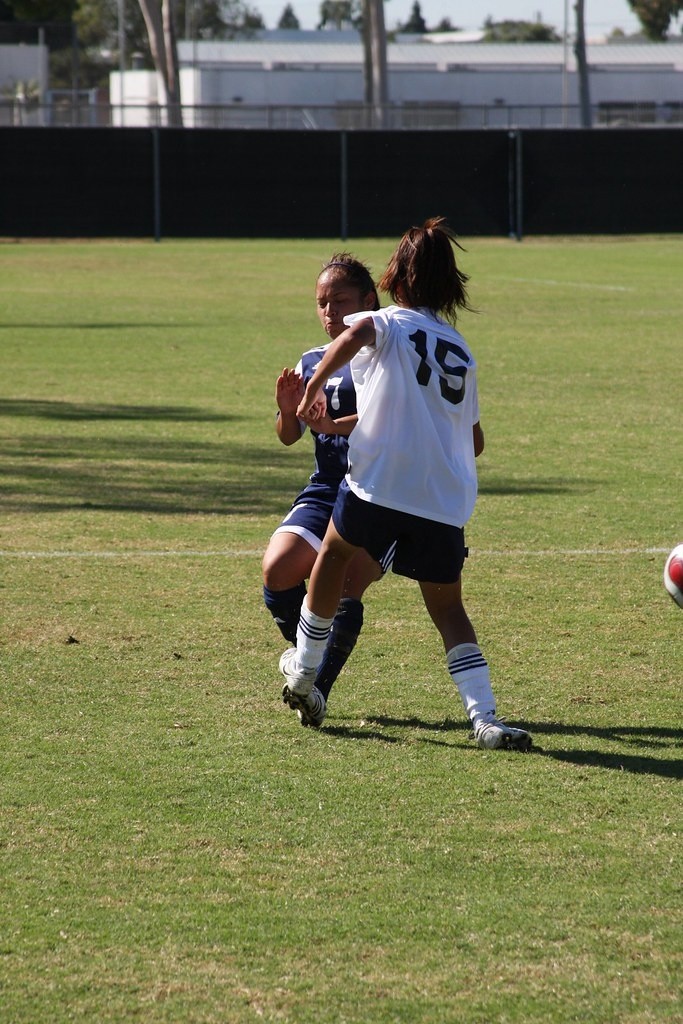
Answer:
[664,541,683,613]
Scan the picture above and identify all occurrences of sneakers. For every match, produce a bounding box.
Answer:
[281,683,327,728]
[279,647,317,699]
[476,723,533,752]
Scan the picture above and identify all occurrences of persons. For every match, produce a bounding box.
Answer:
[261,252,397,727]
[278,217,538,750]
